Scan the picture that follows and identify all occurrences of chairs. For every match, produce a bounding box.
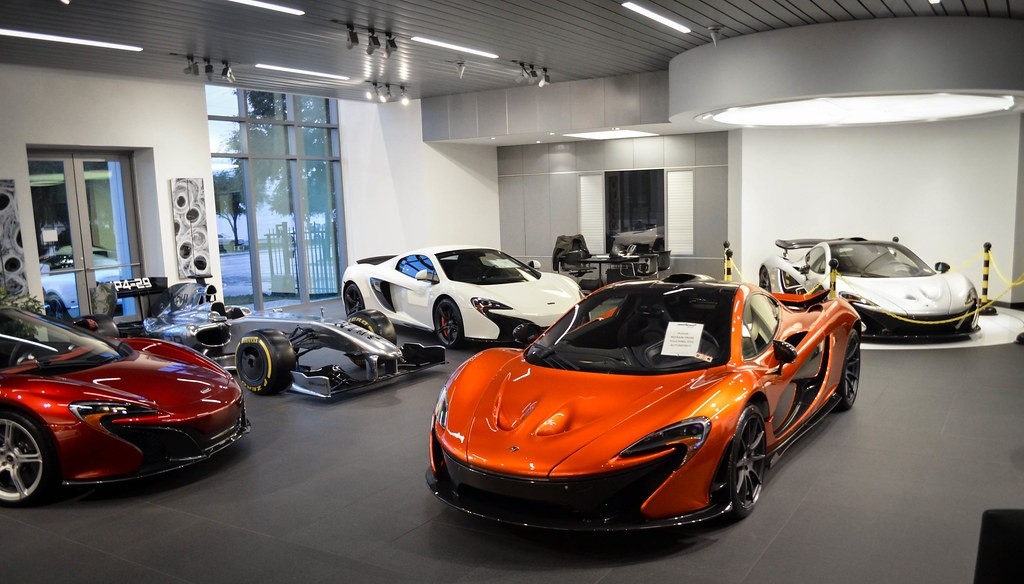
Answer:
[620,250,671,279]
[556,235,597,277]
[617,303,674,347]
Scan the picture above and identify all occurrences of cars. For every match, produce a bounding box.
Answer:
[218,233,244,247]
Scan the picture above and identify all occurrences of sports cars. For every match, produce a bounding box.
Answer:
[34,248,130,325]
[422,273,863,541]
[758,236,982,338]
[340,246,593,351]
[0,305,253,506]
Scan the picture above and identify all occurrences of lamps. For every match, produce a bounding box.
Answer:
[515,63,550,88]
[183,55,236,84]
[366,82,409,106]
[345,28,397,59]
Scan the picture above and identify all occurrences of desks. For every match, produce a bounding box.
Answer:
[577,255,638,287]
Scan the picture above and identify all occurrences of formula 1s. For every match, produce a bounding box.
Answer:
[144,299,451,394]
